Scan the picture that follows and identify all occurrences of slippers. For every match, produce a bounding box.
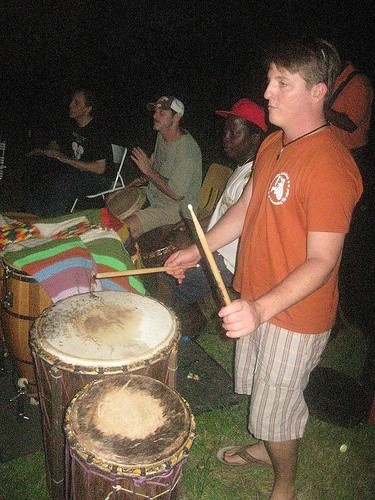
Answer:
[216,445,274,467]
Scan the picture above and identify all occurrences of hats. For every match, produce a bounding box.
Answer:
[147,96,184,117]
[215,98,268,135]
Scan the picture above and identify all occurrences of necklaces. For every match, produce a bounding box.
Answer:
[276,120,330,160]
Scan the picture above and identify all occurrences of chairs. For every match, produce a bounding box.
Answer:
[198,163,233,223]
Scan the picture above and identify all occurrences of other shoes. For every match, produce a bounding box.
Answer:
[184,318,207,340]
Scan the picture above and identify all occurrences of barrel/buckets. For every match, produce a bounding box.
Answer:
[0,258,53,395]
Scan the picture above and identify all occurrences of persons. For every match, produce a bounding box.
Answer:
[150,100,273,351]
[27,87,115,219]
[164,38,364,500]
[105,96,203,249]
[324,40,374,329]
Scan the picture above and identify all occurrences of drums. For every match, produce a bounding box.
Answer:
[107,187,151,223]
[0,242,140,401]
[28,290,182,500]
[136,224,193,271]
[62,373,197,500]
